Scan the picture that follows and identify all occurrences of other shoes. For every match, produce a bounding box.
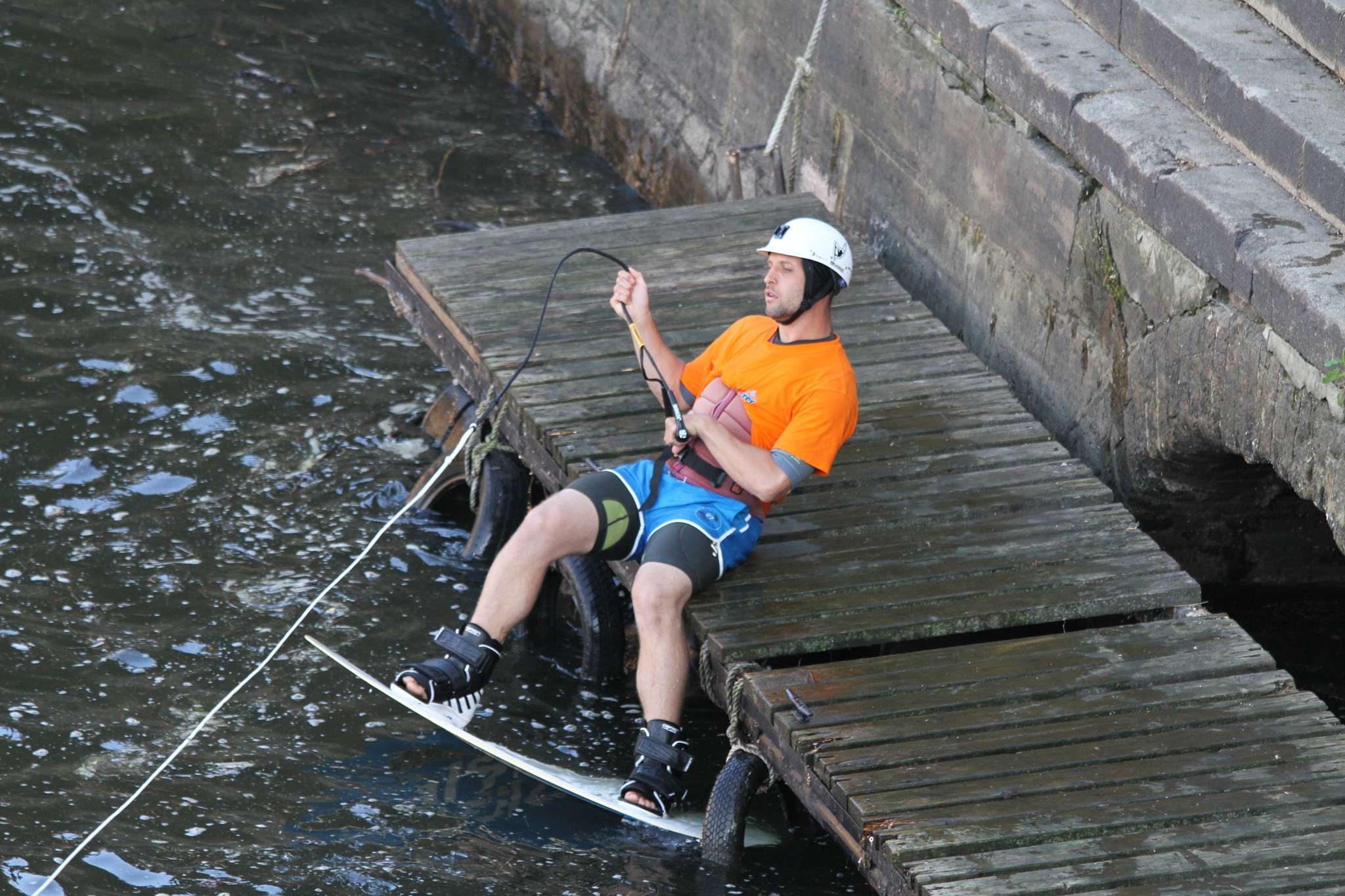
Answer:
[616,716,694,821]
[390,621,504,729]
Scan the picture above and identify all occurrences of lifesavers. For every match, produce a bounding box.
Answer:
[702,749,797,867]
[407,451,531,561]
[515,549,626,683]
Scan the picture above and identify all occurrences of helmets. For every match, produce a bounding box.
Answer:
[756,218,853,286]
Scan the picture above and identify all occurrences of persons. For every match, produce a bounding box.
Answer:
[390,217,859,815]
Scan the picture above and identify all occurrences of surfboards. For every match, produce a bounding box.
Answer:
[306,632,781,849]
